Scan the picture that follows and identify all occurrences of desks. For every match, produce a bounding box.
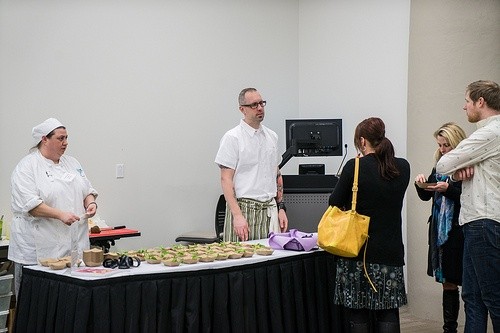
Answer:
[11,238,379,333]
[0,228,141,257]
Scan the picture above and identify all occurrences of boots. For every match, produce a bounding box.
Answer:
[442,288,460,333]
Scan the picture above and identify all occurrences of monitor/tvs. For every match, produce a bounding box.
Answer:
[299,164,325,175]
[285,119,343,157]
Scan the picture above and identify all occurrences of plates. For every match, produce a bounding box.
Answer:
[416,183,437,188]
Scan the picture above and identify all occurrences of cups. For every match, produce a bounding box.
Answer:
[0,220,3,240]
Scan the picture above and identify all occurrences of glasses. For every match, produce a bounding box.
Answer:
[242,101,266,110]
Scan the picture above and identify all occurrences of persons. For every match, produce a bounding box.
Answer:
[414,121,475,333]
[8,117,99,311]
[435,81,500,332]
[214,88,288,243]
[328,117,410,332]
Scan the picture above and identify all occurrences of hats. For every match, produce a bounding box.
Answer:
[31,118,64,146]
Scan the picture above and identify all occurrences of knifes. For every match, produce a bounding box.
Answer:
[100,226,126,231]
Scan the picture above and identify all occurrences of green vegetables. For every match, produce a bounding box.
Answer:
[117,240,265,261]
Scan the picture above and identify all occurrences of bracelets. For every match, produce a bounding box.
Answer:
[276,202,288,211]
[84,201,99,209]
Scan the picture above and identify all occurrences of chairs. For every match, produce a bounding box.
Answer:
[175,194,226,244]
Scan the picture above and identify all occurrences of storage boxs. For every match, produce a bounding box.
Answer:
[0,273,14,333]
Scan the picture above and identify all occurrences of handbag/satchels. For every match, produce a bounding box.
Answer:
[316,205,372,258]
